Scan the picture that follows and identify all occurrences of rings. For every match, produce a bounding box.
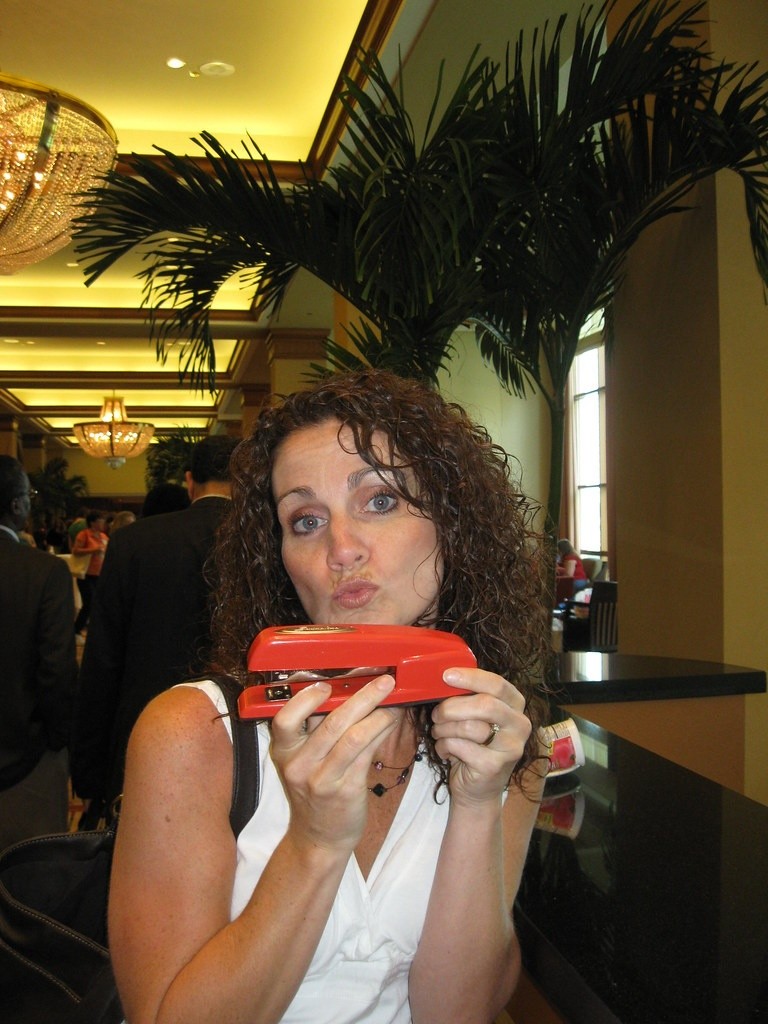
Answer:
[479,723,499,745]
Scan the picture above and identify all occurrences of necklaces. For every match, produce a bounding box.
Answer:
[367,742,427,797]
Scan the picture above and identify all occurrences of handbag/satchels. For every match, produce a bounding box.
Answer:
[55,531,92,580]
[0,675,260,1024]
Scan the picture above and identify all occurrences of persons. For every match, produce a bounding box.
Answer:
[17,485,190,647]
[557,540,587,600]
[69,433,241,829]
[108,369,568,1024]
[0,456,75,850]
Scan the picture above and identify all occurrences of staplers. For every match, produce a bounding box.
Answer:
[236,624,479,719]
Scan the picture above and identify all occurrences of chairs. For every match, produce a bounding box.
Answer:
[561,581,620,653]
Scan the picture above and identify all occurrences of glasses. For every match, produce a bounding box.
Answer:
[10,488,38,499]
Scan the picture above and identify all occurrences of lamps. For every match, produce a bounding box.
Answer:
[0,72,122,280]
[75,390,154,470]
